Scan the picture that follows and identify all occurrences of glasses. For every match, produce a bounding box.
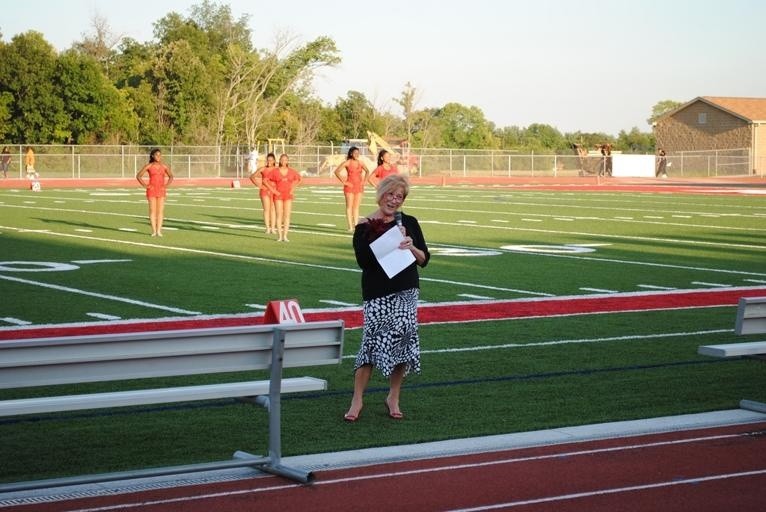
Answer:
[384,192,404,204]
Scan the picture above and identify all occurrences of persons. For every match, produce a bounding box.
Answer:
[136,149,175,237]
[367,150,400,189]
[655,148,668,177]
[262,153,305,242]
[23,146,36,178]
[248,154,279,236]
[0,146,12,178]
[343,174,431,422]
[597,144,613,176]
[333,147,370,232]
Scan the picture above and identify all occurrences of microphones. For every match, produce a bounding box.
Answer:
[395,212,403,226]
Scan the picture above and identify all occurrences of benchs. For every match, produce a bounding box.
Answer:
[693,284,766,415]
[0,315,350,500]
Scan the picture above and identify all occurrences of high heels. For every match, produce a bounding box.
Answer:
[384,398,403,418]
[344,407,364,422]
